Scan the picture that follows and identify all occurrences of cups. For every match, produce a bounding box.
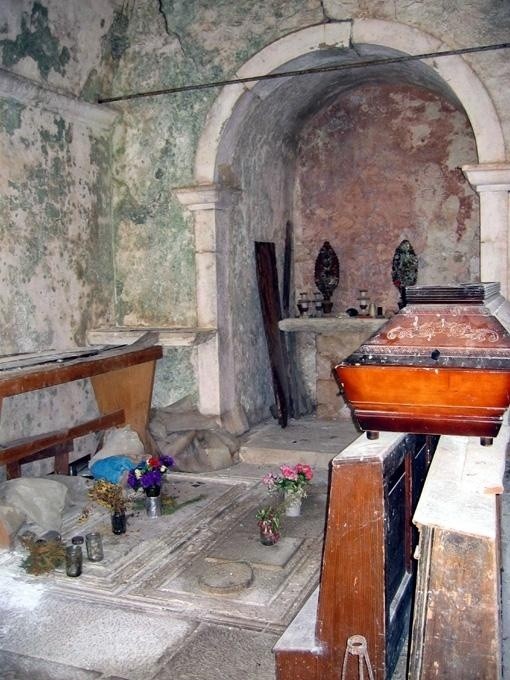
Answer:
[146,496,162,517]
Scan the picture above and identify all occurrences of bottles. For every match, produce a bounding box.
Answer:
[86,533,104,562]
[369,303,376,317]
[65,546,82,576]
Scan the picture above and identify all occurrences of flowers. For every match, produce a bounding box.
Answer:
[126,453,177,491]
[88,479,129,514]
[254,507,284,543]
[262,462,314,497]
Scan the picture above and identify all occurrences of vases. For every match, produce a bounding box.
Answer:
[144,486,163,520]
[260,531,279,546]
[283,491,301,517]
